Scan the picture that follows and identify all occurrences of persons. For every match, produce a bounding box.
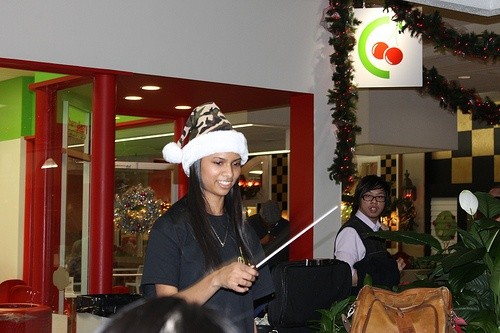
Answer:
[67,231,82,278]
[333,174,407,297]
[142,100,276,333]
[248,202,288,264]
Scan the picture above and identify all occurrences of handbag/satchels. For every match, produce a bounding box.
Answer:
[268,255,352,332]
[341,285,459,333]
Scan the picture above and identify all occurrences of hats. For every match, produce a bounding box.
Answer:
[162,102,249,177]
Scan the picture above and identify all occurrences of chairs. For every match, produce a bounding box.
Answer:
[125,265,144,293]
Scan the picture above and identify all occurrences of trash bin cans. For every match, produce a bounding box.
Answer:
[76,293,143,332]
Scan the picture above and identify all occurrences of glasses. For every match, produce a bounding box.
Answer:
[361,195,386,202]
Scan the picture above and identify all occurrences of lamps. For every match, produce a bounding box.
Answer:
[40,158,58,169]
[402,168,417,201]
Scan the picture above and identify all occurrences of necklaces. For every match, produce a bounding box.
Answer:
[204,206,236,248]
